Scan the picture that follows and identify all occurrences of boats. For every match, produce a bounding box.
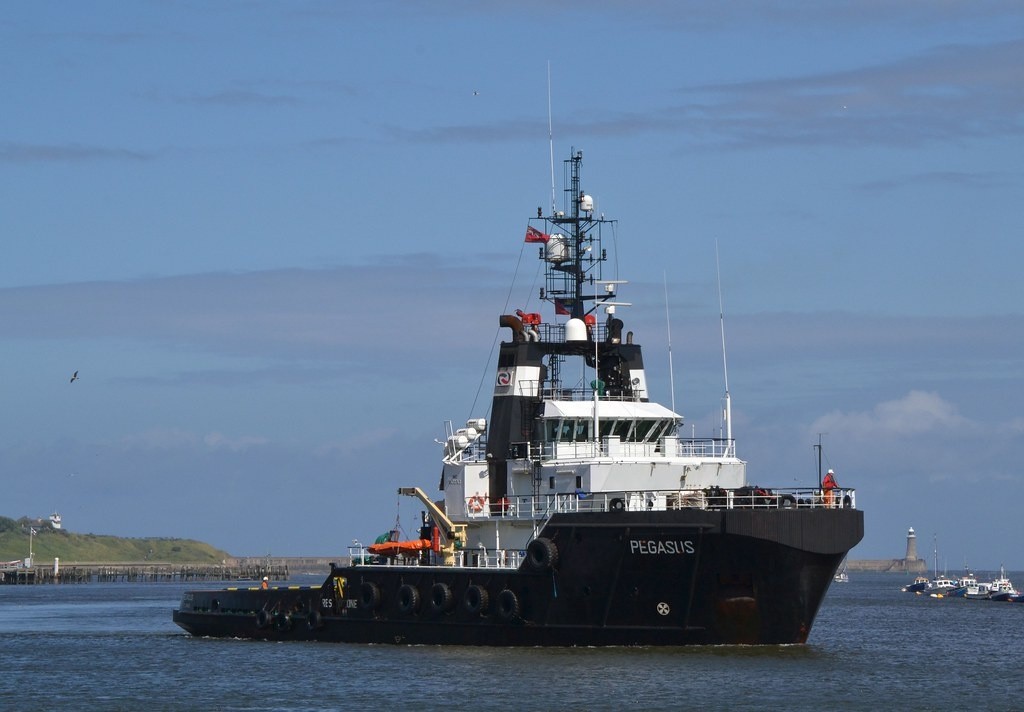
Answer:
[367,538,431,557]
[834,571,849,582]
[905,530,1024,603]
[172,58,865,645]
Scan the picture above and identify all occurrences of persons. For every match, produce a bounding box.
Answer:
[262,577,269,589]
[823,469,840,509]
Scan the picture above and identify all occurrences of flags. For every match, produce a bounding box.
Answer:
[525,226,551,244]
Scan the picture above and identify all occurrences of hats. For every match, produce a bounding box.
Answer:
[828,469,834,473]
[263,577,268,580]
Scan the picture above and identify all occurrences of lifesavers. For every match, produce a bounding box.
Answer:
[304,610,322,631]
[493,589,520,623]
[462,584,488,615]
[355,581,381,611]
[394,585,421,613]
[255,610,271,630]
[426,583,453,614]
[469,496,485,511]
[526,536,559,572]
